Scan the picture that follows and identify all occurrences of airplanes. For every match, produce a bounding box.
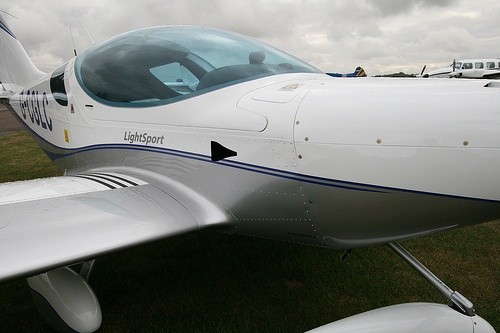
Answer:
[0,7,500,333]
[416,59,500,78]
